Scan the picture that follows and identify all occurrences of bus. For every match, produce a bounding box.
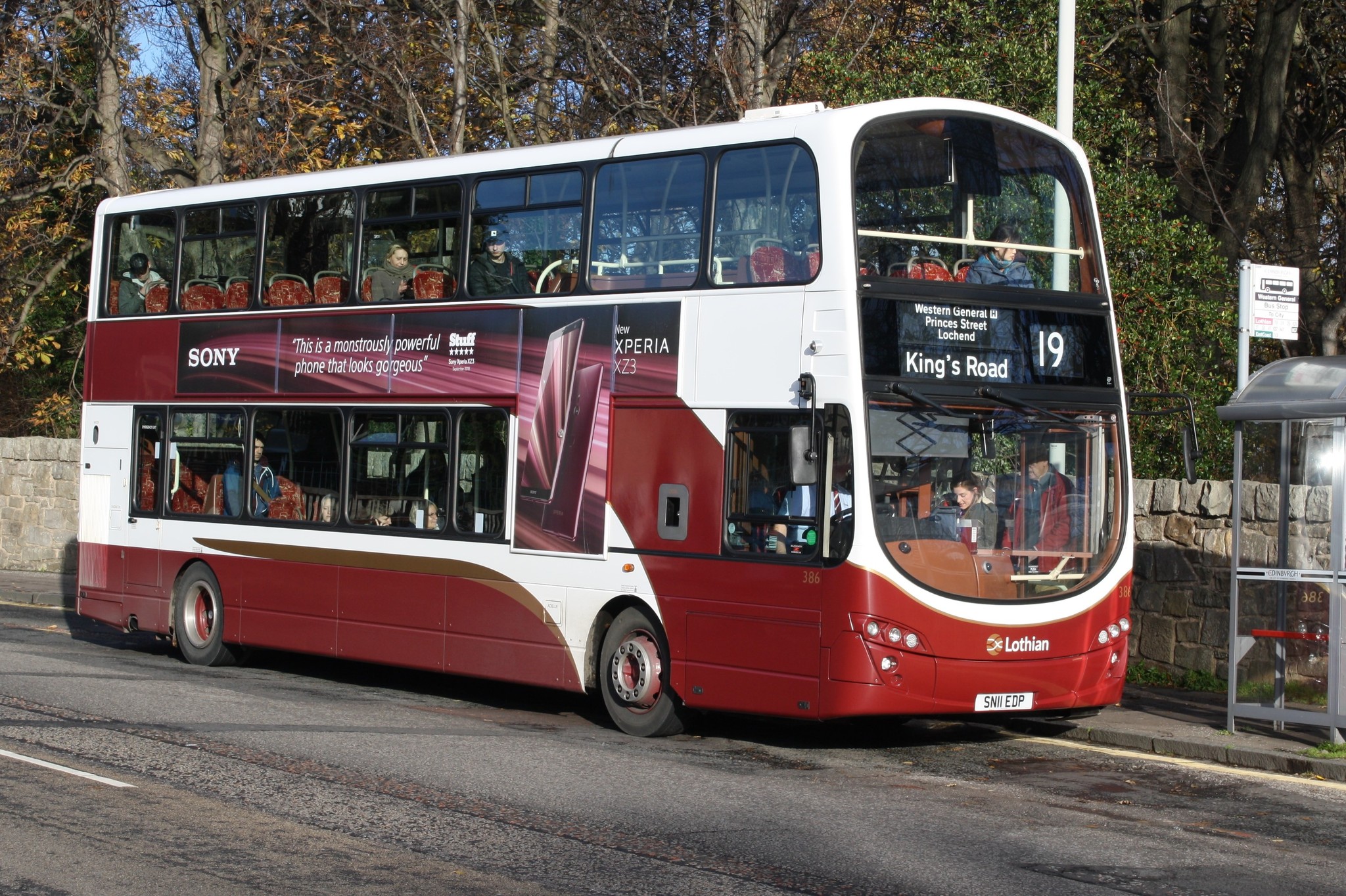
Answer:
[76,95,1202,739]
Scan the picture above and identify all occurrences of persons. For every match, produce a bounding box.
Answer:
[371,240,419,302]
[939,472,998,549]
[770,445,852,554]
[469,224,535,297]
[320,439,506,532]
[998,445,1079,596]
[221,432,282,518]
[966,224,1035,289]
[118,253,169,315]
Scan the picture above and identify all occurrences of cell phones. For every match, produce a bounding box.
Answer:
[519,319,603,542]
[407,278,413,287]
[370,517,382,526]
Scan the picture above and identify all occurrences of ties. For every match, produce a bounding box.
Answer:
[832,487,843,520]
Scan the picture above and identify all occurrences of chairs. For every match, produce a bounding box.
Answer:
[225,276,268,309]
[183,279,224,311]
[760,484,796,553]
[171,456,214,513]
[953,258,977,282]
[145,281,184,313]
[749,237,804,282]
[886,263,908,278]
[800,243,819,280]
[268,274,315,307]
[109,280,119,315]
[201,474,224,515]
[139,437,156,510]
[268,475,307,520]
[859,259,881,276]
[313,270,351,304]
[524,264,555,293]
[412,264,457,300]
[362,266,382,301]
[907,257,954,282]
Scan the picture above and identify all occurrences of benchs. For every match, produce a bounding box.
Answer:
[533,255,749,294]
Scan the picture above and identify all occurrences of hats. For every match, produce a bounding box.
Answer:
[482,224,510,242]
[130,253,149,269]
[1025,436,1049,465]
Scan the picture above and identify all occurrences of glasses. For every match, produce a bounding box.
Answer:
[486,239,505,246]
[130,267,147,274]
[427,513,437,518]
